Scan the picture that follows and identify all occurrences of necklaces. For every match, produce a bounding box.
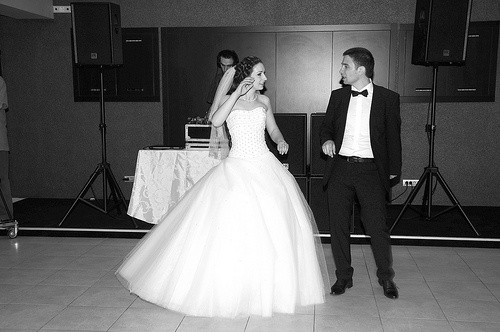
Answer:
[242,95,258,103]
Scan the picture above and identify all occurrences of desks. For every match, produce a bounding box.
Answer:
[127,150,229,224]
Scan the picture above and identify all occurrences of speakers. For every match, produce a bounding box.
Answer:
[411,0,472,67]
[70,2,123,65]
[264,113,356,234]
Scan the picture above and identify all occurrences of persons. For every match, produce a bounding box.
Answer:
[319,47,403,299]
[211,56,289,319]
[0,75,18,231]
[206,49,240,148]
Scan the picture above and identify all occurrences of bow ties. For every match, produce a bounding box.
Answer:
[351,89,368,97]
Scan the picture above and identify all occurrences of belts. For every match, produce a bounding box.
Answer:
[330,154,377,164]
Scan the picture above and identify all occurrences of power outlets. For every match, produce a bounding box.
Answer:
[124,176,135,182]
[402,179,419,187]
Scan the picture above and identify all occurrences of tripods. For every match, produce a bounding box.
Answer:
[389,66,480,236]
[58,64,139,229]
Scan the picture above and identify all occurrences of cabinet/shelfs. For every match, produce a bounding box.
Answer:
[70,27,160,102]
[397,20,500,102]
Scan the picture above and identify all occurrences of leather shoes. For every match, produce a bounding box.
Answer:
[378,279,399,299]
[331,276,353,295]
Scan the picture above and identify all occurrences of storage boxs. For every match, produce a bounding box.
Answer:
[185,125,230,151]
